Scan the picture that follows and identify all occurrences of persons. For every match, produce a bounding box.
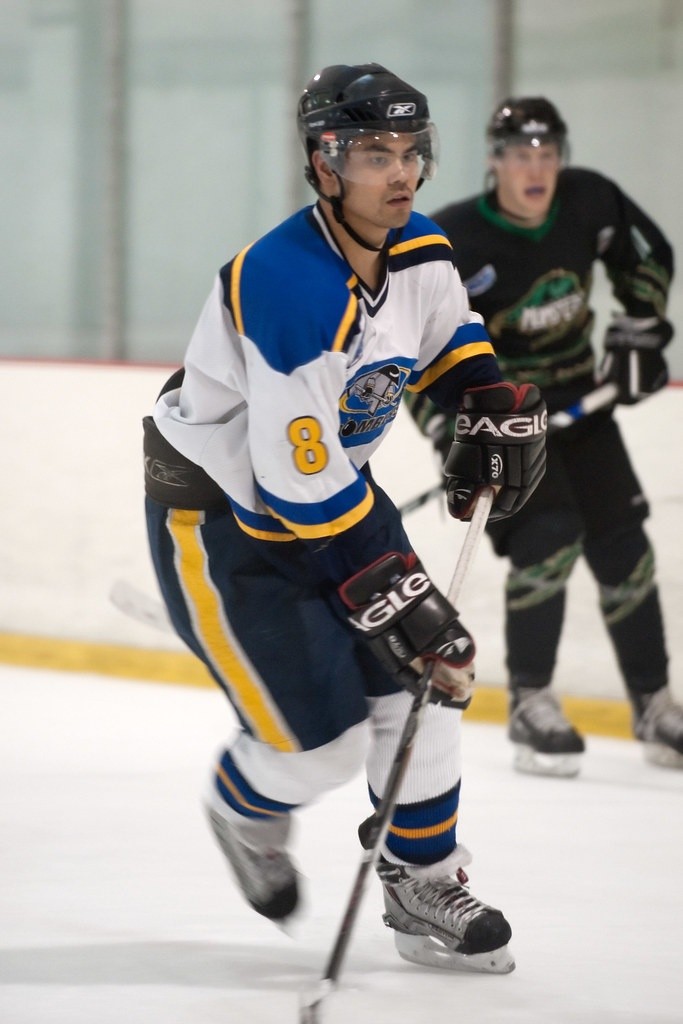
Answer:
[141,61,548,975]
[401,96,683,777]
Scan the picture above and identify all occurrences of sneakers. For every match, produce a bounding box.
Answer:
[209,798,306,946]
[509,685,585,777]
[634,683,683,767]
[358,824,516,974]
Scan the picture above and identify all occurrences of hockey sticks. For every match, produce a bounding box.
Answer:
[301,482,509,1024]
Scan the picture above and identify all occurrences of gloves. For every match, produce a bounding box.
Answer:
[338,552,476,709]
[433,419,455,465]
[605,311,673,405]
[444,384,548,522]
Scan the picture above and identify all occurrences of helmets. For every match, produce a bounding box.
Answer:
[297,63,430,155]
[485,98,567,153]
